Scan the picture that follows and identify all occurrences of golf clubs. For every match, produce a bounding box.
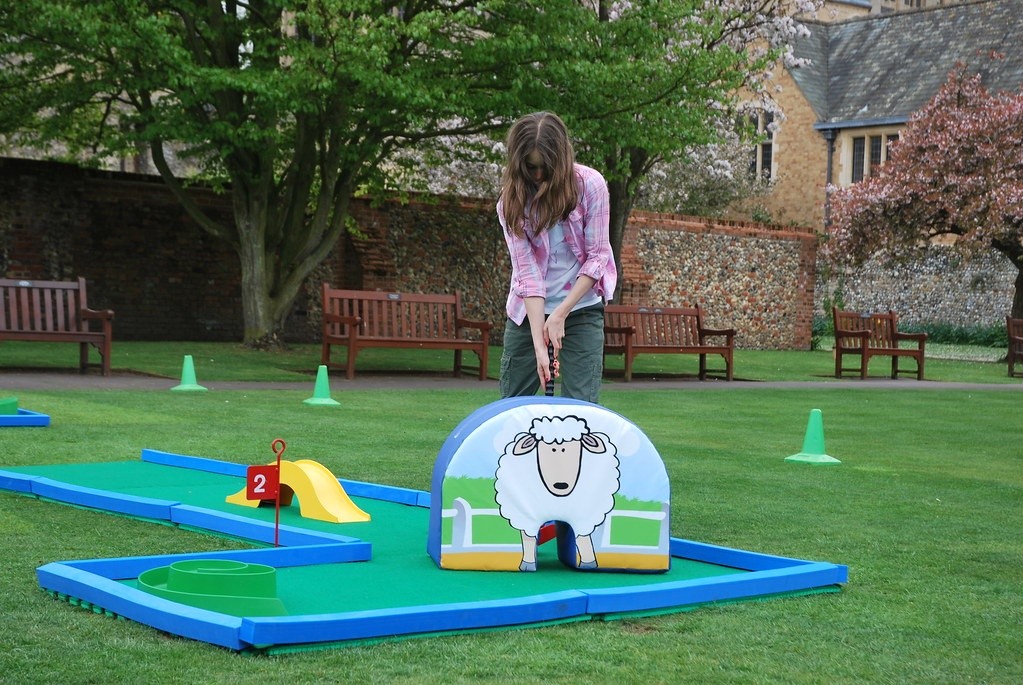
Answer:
[538,338,557,546]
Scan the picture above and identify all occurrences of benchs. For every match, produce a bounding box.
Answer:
[1006,316,1023,378]
[0,277,115,376]
[832,306,929,380]
[602,303,737,382]
[320,283,494,381]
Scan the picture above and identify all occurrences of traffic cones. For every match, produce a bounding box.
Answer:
[783,409,842,465]
[169,355,208,391]
[302,365,342,406]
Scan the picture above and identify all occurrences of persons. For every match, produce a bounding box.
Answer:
[495,110,617,403]
